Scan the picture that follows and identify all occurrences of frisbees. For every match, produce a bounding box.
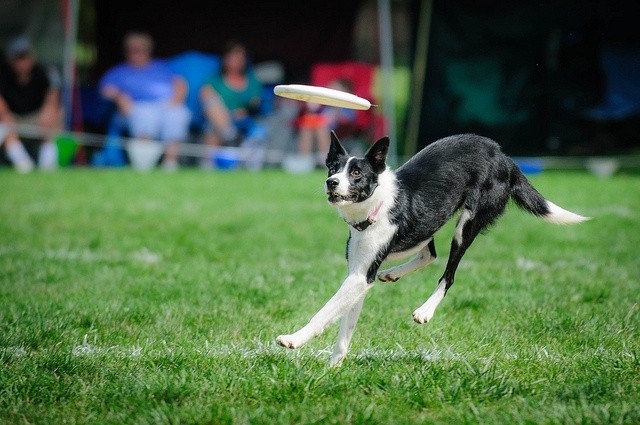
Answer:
[274,84,371,110]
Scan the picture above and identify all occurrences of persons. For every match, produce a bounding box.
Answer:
[199,39,269,171]
[1,36,63,173]
[97,31,192,171]
[292,75,357,168]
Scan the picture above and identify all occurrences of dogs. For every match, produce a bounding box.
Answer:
[276,129,594,368]
